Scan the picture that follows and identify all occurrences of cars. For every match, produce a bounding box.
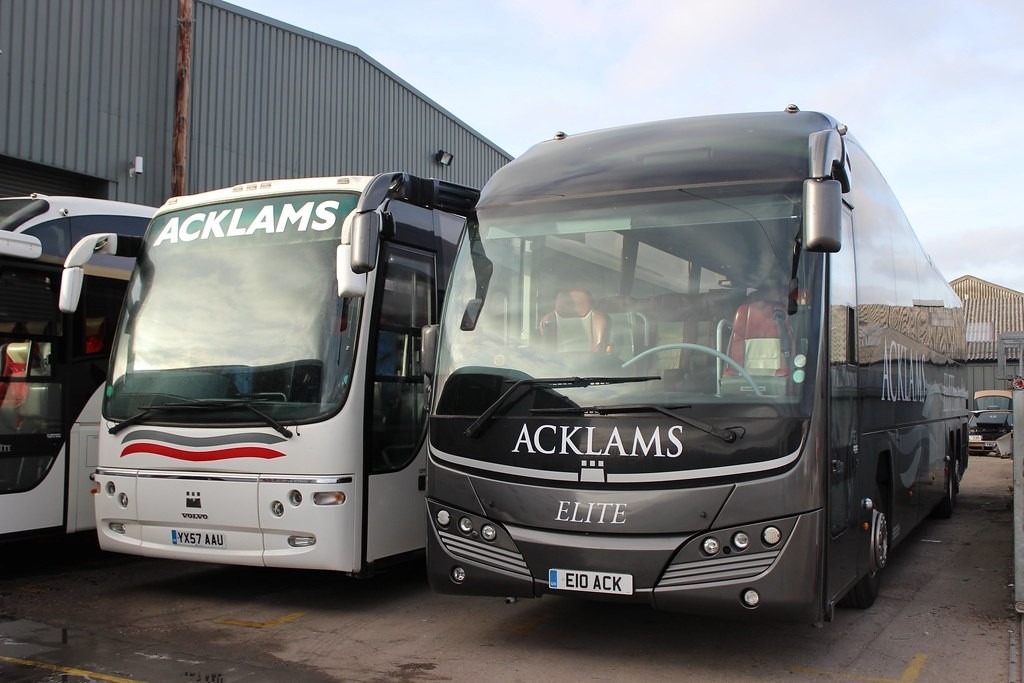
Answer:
[967,411,1013,455]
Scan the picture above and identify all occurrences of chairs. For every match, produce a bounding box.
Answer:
[542,286,813,391]
[0,341,53,426]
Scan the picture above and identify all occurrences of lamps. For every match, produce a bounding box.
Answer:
[435,150,453,166]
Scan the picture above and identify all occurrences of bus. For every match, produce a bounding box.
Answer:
[59,172,712,574]
[426,103,970,623]
[0,194,158,535]
